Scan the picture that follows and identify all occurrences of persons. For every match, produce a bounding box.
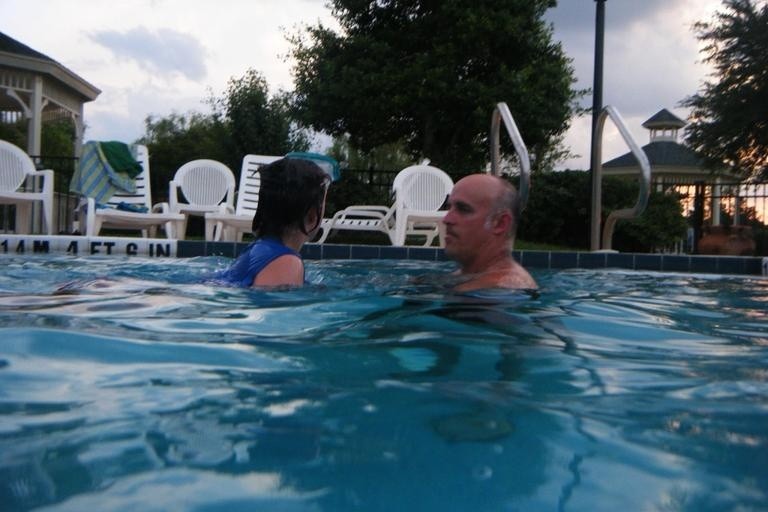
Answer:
[408,173,538,295]
[198,156,332,289]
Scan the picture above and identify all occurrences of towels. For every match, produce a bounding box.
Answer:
[70,140,138,206]
[100,142,143,181]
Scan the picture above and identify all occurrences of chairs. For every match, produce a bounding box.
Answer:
[393,165,455,247]
[304,157,432,245]
[169,159,236,242]
[0,140,54,235]
[74,139,185,237]
[205,154,285,242]
